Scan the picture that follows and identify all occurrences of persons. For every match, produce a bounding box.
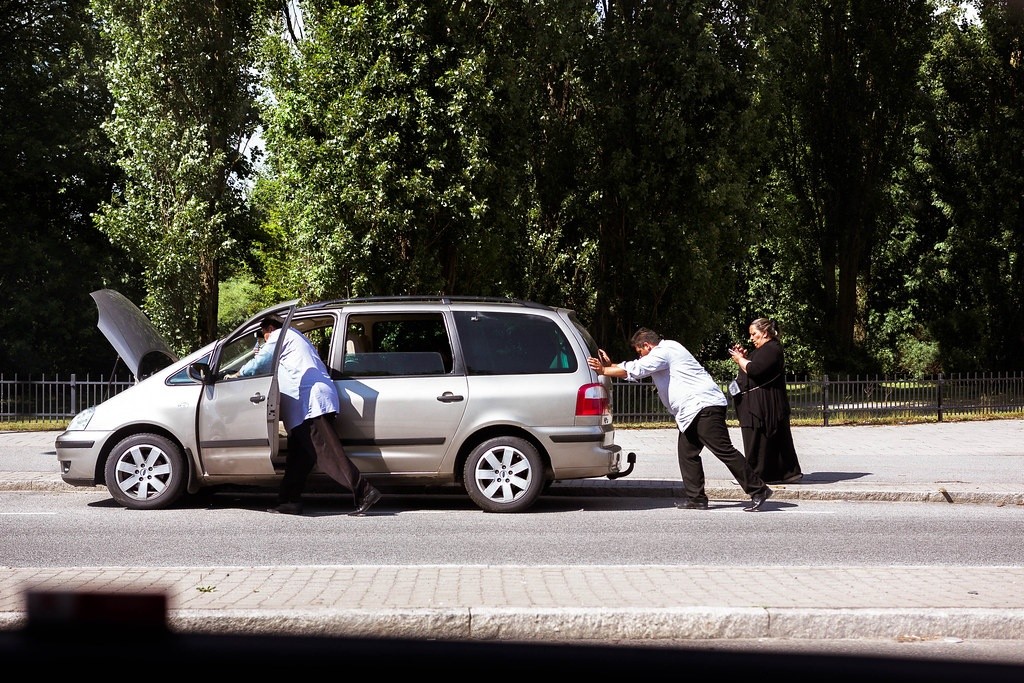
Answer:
[585,328,774,513]
[221,314,384,517]
[729,317,804,485]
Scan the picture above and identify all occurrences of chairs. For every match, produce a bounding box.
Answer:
[346,333,369,360]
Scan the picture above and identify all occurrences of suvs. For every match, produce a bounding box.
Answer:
[54,288,636,511]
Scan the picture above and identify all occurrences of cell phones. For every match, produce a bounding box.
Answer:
[729,349,735,354]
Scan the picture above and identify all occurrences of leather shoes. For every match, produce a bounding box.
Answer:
[673,500,708,509]
[265,504,303,515]
[743,487,773,512]
[348,487,383,516]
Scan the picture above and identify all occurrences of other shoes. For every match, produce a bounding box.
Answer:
[766,473,803,485]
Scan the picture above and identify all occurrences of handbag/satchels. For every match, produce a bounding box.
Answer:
[728,380,742,401]
